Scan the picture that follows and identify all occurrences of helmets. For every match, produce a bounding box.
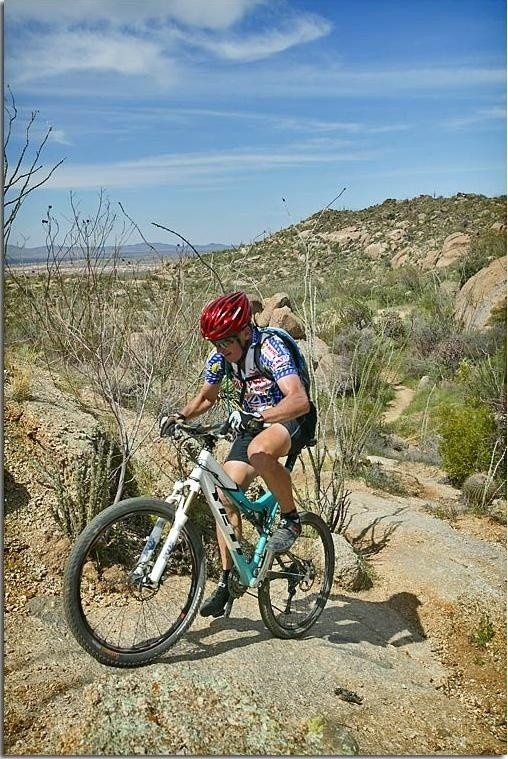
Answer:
[198,291,252,341]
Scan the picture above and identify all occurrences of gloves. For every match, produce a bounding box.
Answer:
[228,411,264,433]
[159,412,186,438]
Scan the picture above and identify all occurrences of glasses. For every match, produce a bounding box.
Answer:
[212,340,233,349]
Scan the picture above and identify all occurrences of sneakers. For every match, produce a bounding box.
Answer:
[265,515,302,552]
[200,587,230,617]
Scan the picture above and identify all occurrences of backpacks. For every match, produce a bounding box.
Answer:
[254,327,310,394]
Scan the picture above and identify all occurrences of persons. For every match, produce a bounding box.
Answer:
[159,291,317,617]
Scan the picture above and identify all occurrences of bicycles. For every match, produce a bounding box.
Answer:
[63,416,335,668]
